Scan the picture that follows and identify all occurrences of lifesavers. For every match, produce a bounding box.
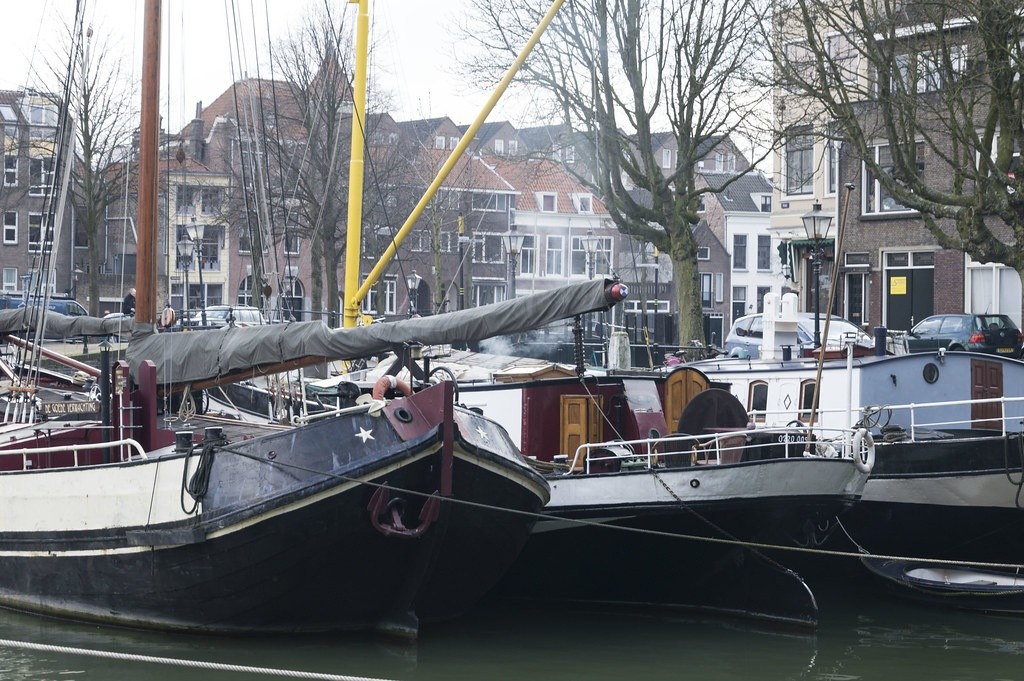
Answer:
[852,427,877,473]
[372,374,416,402]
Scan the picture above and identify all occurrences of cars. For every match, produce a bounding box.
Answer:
[896,314,1023,358]
[1,295,89,318]
[723,311,895,356]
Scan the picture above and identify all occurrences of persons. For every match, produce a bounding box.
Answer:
[123,288,136,315]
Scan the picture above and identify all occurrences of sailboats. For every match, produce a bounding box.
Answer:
[0,4,1023,642]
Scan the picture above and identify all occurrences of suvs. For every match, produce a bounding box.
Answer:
[175,305,265,329]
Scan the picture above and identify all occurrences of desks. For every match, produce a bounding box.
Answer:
[719,433,817,464]
